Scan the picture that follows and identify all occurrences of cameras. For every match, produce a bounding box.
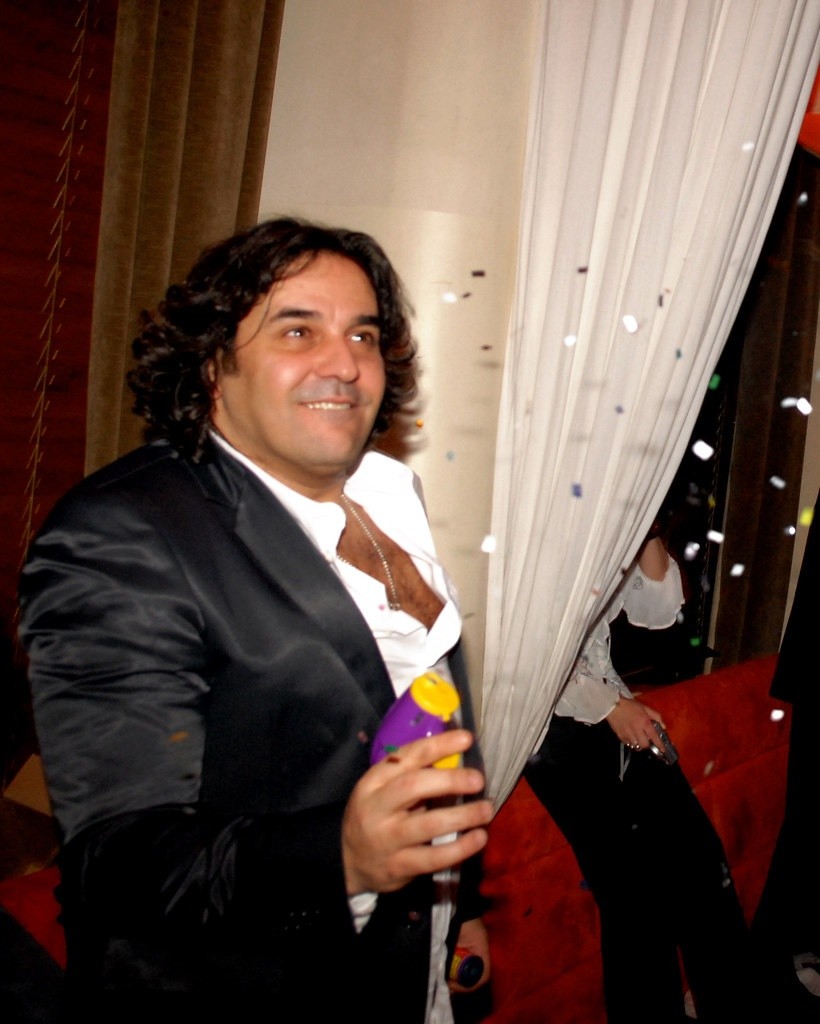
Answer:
[647,721,679,765]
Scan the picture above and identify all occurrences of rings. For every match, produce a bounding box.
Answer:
[629,742,638,750]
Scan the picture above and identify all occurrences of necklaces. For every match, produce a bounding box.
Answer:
[316,490,402,610]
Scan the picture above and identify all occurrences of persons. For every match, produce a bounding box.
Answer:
[25,213,498,1024]
[530,531,756,1024]
